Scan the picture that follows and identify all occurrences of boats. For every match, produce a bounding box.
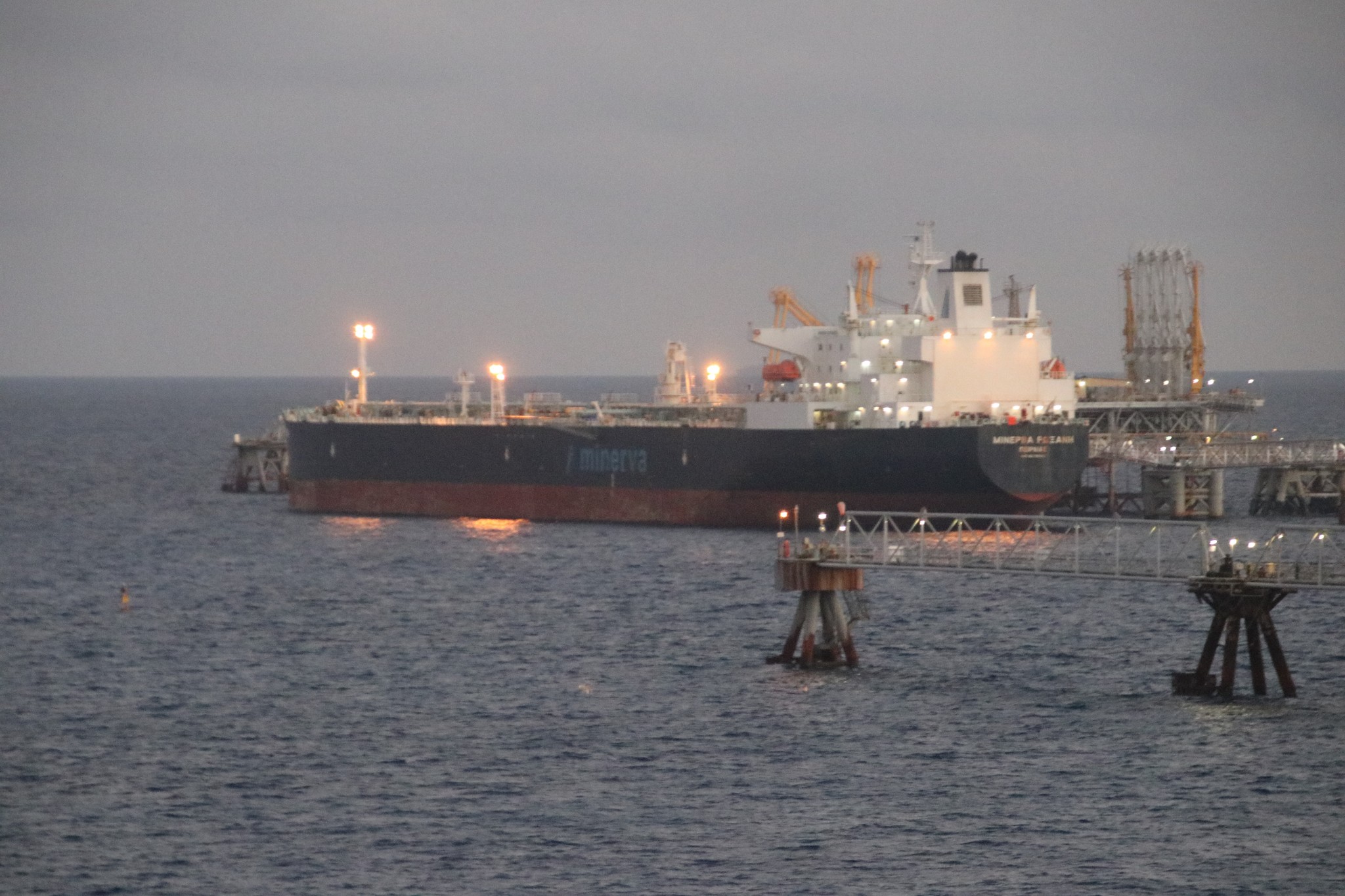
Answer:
[761,360,803,383]
[278,216,1094,536]
